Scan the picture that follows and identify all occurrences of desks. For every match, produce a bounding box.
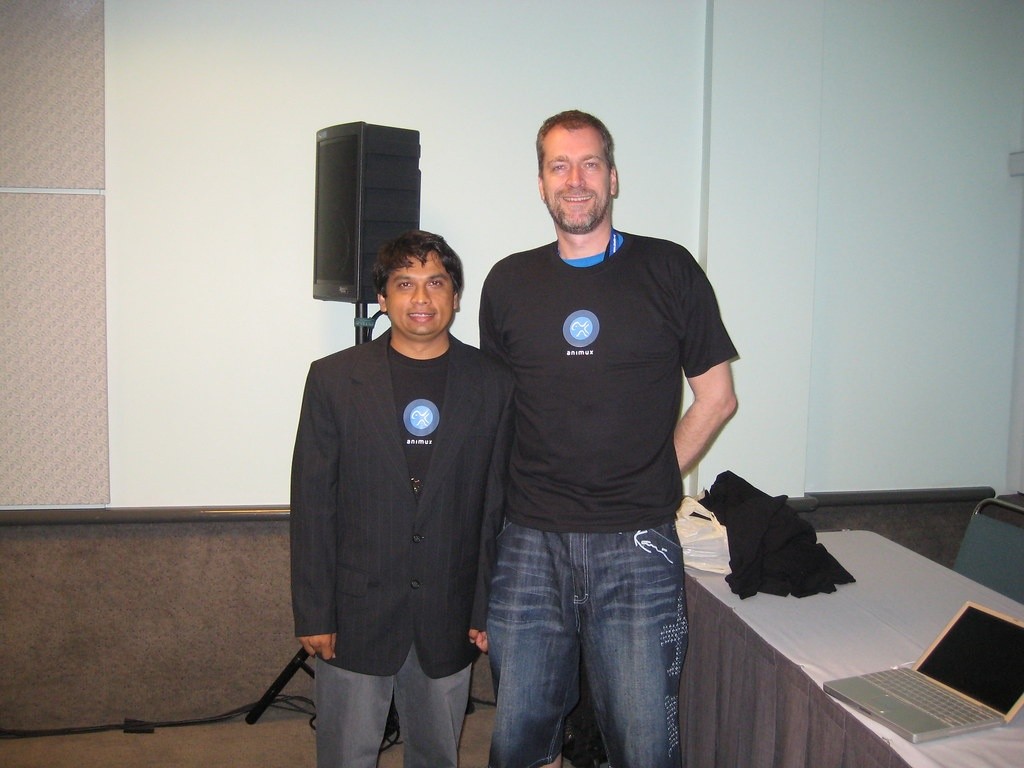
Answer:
[678,527,1024,768]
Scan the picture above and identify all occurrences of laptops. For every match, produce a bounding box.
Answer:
[823,601,1024,743]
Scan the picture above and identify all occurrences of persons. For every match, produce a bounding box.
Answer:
[291,231,519,768]
[478,110,740,768]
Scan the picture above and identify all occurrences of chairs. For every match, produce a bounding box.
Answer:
[954,497,1024,606]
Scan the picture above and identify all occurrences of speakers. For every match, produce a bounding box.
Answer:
[314,121,421,303]
